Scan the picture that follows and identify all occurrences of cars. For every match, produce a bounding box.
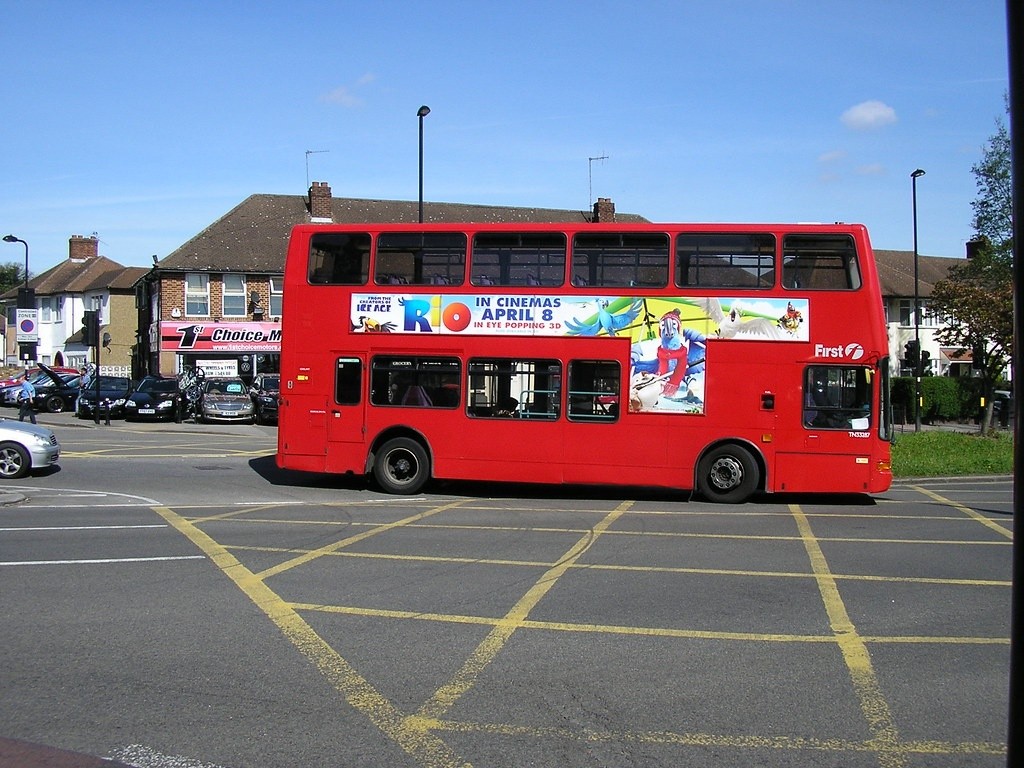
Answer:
[77,375,136,420]
[0,363,81,413]
[198,376,255,425]
[123,373,190,423]
[0,417,62,479]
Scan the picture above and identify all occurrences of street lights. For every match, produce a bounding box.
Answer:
[3,235,29,380]
[910,167,926,438]
[413,105,432,223]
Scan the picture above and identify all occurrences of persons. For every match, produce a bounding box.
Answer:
[184,365,195,419]
[494,396,518,418]
[20,375,36,424]
[73,367,90,417]
[812,372,851,427]
[605,404,618,416]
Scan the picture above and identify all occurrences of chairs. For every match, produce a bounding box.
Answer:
[311,264,588,287]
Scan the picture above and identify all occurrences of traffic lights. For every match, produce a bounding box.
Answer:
[904,340,917,367]
[922,350,930,370]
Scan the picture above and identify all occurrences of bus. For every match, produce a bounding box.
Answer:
[274,218,894,503]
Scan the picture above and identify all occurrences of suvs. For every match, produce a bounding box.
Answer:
[248,372,280,426]
